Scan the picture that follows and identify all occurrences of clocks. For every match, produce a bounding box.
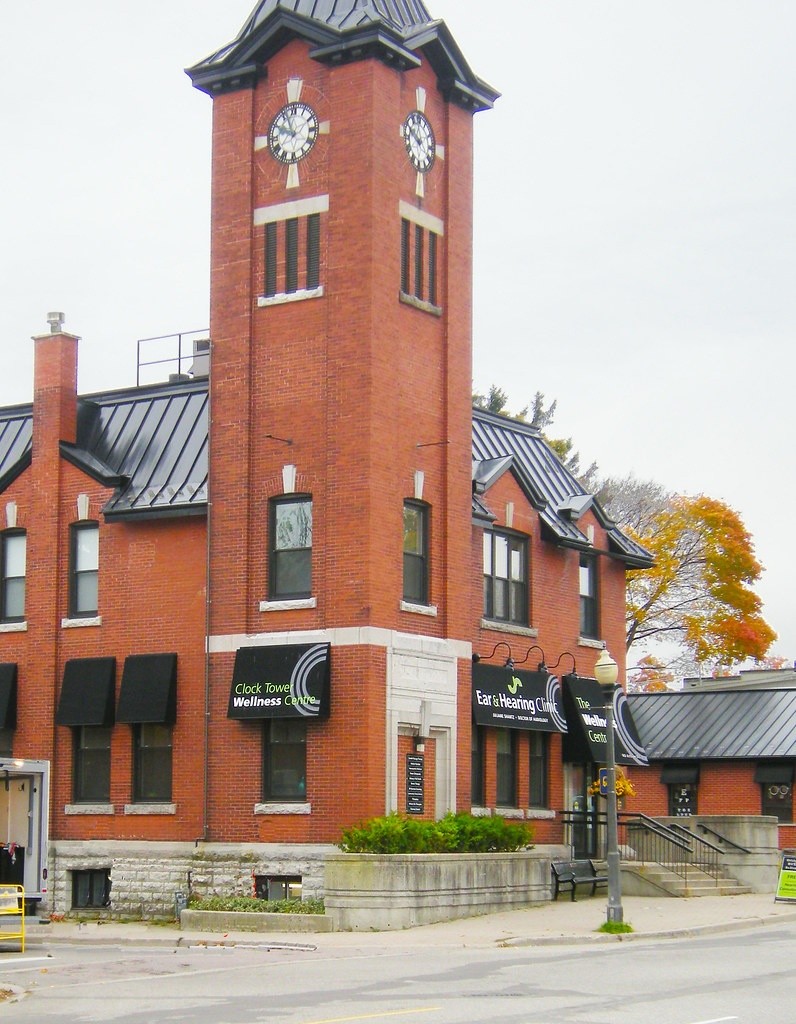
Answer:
[403,111,436,172]
[267,103,319,164]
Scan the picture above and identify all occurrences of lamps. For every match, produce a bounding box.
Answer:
[472,643,514,670]
[512,646,548,670]
[539,652,579,678]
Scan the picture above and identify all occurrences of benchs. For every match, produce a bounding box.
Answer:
[551,859,608,902]
